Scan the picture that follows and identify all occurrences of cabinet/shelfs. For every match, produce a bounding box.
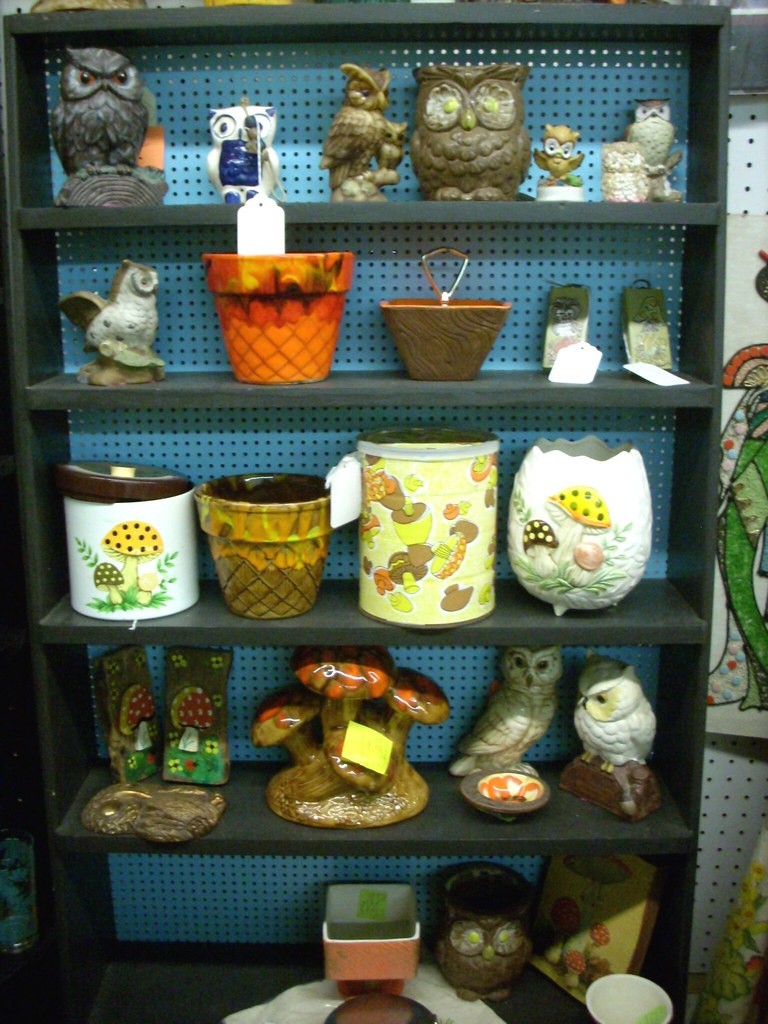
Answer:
[0,3,732,1024]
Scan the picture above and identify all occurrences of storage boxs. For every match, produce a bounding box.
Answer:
[527,848,664,1005]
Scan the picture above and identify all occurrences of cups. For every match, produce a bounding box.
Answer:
[585,973,673,1023]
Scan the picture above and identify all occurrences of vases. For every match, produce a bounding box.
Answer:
[193,472,333,621]
[505,433,655,617]
[202,251,354,385]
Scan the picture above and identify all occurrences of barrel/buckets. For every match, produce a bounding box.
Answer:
[202,252,355,385]
[195,474,331,619]
